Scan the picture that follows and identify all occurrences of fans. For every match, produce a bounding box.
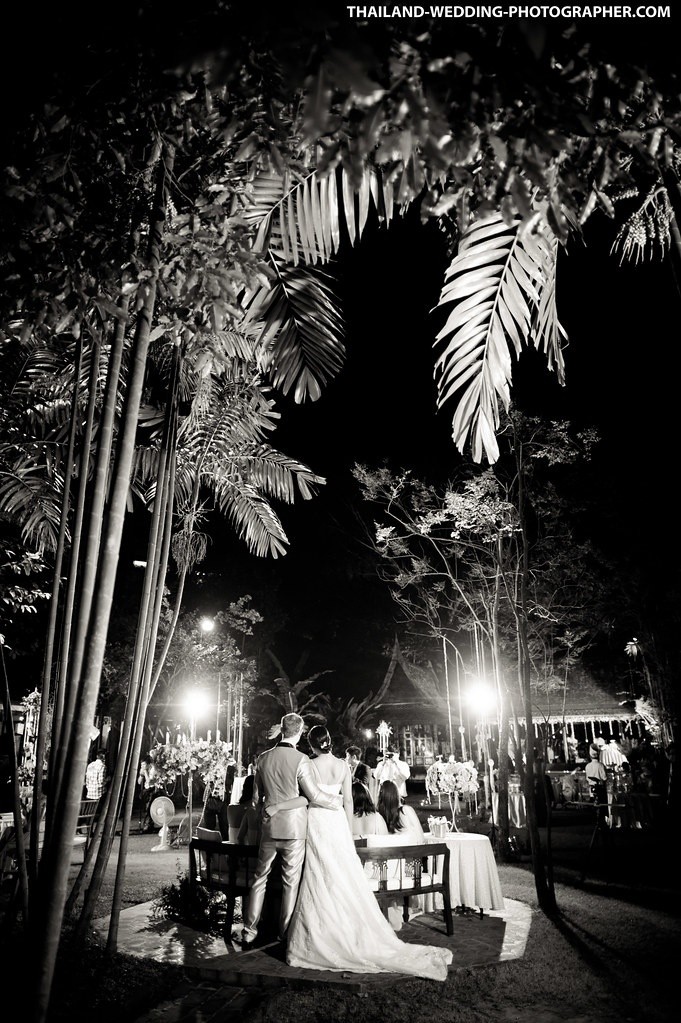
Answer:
[150,796,175,852]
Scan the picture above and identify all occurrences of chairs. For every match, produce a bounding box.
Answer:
[70,799,99,865]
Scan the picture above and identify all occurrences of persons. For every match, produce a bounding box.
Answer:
[585,745,608,801]
[85,749,110,801]
[207,712,453,981]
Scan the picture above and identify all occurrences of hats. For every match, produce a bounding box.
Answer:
[589,744,599,758]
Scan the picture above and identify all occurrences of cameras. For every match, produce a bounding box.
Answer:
[386,753,394,758]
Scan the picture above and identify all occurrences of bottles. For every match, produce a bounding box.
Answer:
[428,814,447,837]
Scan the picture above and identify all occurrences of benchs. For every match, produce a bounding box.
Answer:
[188,837,454,946]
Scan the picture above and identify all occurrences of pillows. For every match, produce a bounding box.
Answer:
[195,826,229,872]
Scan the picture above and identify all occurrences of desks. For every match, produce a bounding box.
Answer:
[419,832,504,920]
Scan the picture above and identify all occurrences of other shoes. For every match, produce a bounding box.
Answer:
[243,940,253,950]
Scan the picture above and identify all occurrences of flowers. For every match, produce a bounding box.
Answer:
[139,737,236,792]
[422,758,480,796]
